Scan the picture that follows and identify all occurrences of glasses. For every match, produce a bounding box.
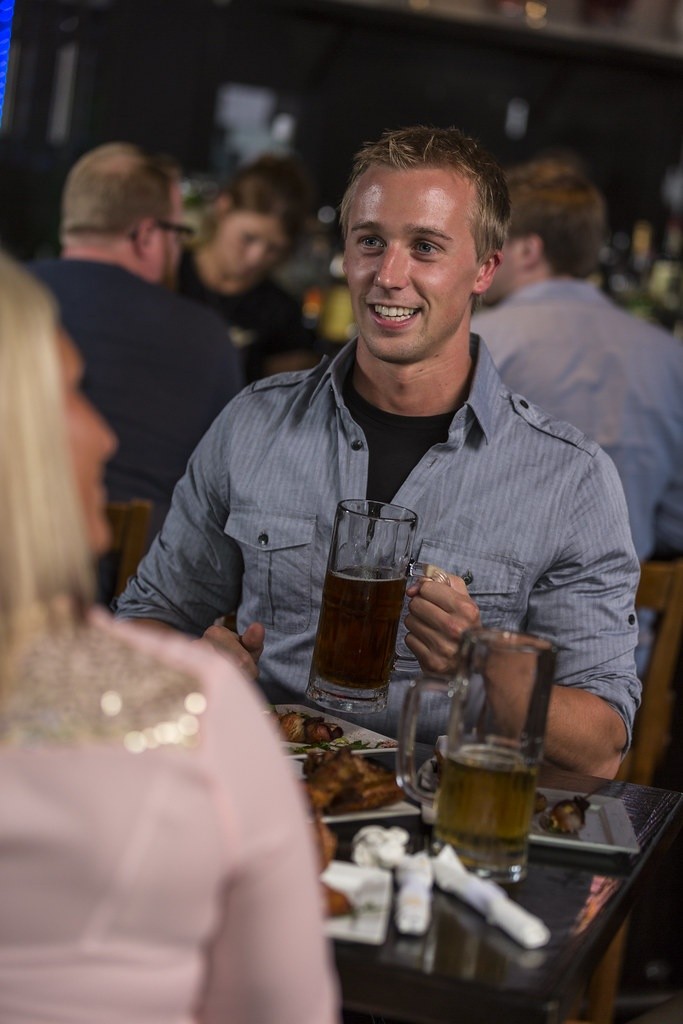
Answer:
[129,219,195,243]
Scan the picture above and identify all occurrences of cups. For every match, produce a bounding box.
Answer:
[304,499,451,713]
[396,628,556,883]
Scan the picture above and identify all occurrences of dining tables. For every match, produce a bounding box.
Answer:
[318,765,683,1024]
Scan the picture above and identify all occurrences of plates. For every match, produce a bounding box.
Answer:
[272,705,413,759]
[297,761,421,824]
[422,775,639,856]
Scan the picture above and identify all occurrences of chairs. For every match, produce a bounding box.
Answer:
[568,561,683,1024]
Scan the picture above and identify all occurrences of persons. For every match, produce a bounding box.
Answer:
[115,121,643,780]
[469,159,683,675]
[25,140,240,606]
[174,153,318,384]
[0,249,340,1024]
